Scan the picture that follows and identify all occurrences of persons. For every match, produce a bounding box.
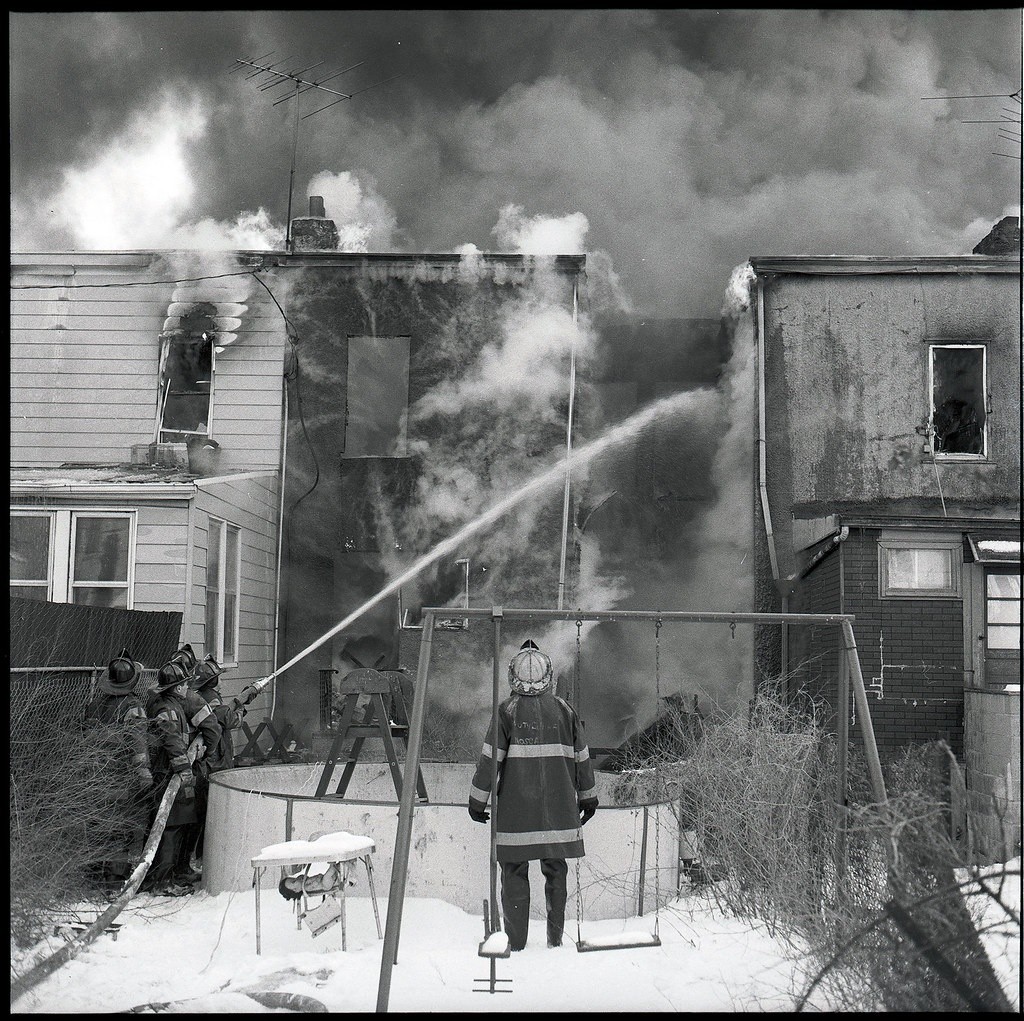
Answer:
[83,649,258,896]
[467,649,598,951]
[279,861,342,901]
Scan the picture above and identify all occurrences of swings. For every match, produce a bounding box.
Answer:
[573,621,661,954]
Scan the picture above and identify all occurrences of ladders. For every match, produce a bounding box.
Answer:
[314,669,428,804]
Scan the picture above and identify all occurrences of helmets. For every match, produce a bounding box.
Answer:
[153,661,193,694]
[170,650,201,674]
[98,657,145,696]
[508,647,553,696]
[191,661,226,689]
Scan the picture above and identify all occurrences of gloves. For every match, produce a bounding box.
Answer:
[234,698,247,717]
[241,686,258,704]
[180,769,194,787]
[469,807,491,824]
[139,778,154,794]
[579,806,595,826]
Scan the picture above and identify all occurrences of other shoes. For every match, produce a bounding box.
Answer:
[176,868,202,882]
[155,885,196,896]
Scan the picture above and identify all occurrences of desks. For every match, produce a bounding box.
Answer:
[250,845,383,955]
